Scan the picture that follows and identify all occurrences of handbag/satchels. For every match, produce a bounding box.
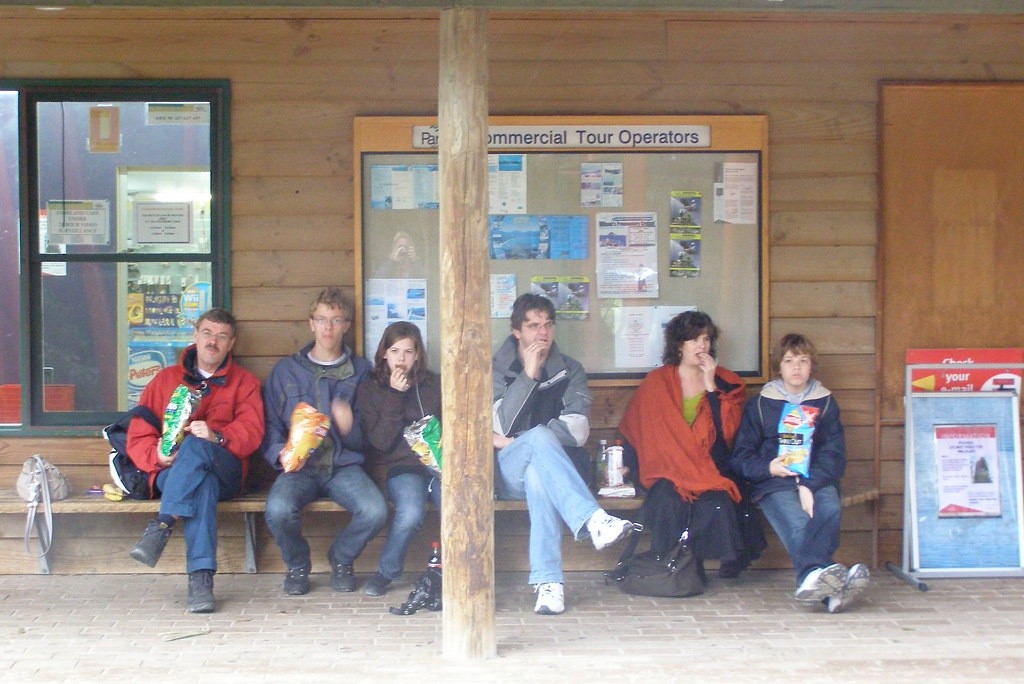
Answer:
[16,454,68,558]
[603,478,704,597]
[102,406,162,500]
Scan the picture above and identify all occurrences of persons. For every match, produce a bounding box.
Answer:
[258,289,387,594]
[357,321,440,595]
[127,308,264,612]
[605,311,768,583]
[732,333,870,614]
[492,293,634,614]
[686,243,696,254]
[686,199,696,210]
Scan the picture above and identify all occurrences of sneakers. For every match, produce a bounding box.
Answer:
[828,563,870,613]
[326,552,356,592]
[129,519,172,568]
[283,563,311,595]
[364,571,390,596]
[187,570,217,613]
[589,507,634,550]
[796,563,848,608]
[534,582,565,615]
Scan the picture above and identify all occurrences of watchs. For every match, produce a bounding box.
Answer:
[214,430,224,444]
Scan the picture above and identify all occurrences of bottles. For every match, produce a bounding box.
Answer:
[427,542,441,568]
[128,238,140,294]
[596,439,607,489]
[139,275,186,294]
[614,440,621,446]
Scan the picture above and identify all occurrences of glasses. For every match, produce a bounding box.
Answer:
[311,315,352,327]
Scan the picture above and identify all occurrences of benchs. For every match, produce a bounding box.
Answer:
[0,488,878,580]
